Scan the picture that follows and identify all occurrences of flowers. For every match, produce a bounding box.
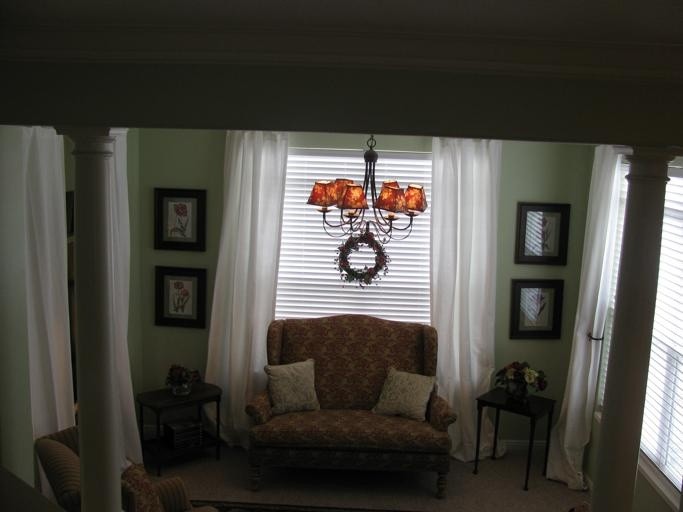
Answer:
[331,235,392,290]
[164,363,194,385]
[493,361,548,393]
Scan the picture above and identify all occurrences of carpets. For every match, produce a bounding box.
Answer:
[188,499,421,512]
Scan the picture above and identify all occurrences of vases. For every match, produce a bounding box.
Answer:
[514,383,528,397]
[172,383,192,396]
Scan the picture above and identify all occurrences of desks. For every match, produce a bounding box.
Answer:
[473,387,557,491]
[137,383,222,476]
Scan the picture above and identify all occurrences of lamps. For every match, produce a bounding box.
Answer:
[305,133,428,245]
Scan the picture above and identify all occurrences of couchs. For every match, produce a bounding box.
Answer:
[238,313,458,500]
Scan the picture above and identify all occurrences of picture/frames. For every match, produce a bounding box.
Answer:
[152,186,207,253]
[514,200,571,268]
[508,278,565,341]
[154,264,207,330]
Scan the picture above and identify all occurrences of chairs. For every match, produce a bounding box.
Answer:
[33,425,219,512]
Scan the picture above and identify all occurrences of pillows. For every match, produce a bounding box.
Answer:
[370,365,438,422]
[262,357,321,415]
